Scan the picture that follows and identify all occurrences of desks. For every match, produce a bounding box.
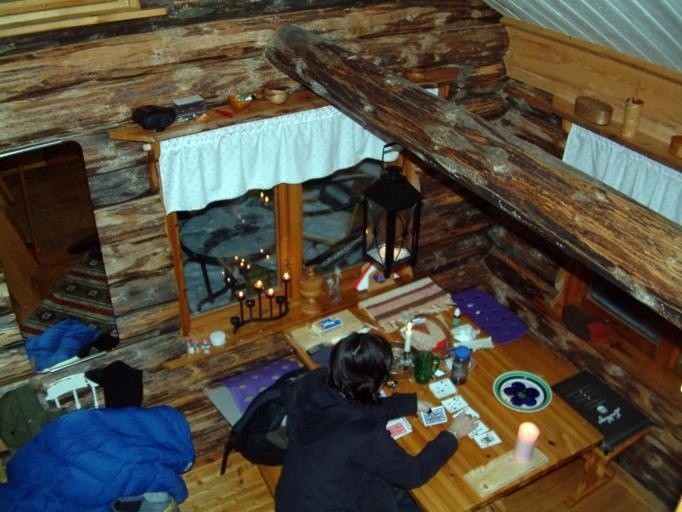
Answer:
[285,275,605,512]
[179,204,276,312]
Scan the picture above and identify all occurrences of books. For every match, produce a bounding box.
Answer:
[309,307,366,342]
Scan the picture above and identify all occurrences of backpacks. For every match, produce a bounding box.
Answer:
[228,367,310,466]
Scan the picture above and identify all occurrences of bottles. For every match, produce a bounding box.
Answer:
[451,307,462,327]
[451,346,471,385]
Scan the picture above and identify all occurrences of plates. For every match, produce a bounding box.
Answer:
[387,342,419,381]
[492,370,553,414]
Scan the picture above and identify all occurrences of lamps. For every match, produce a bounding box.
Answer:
[361,141,426,278]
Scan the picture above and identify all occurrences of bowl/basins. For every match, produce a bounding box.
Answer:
[228,94,253,112]
[264,85,289,104]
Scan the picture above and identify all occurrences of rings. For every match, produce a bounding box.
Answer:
[426,408,433,414]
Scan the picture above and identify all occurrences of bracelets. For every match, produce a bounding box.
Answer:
[447,427,459,441]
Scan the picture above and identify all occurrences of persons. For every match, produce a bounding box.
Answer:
[271,331,480,512]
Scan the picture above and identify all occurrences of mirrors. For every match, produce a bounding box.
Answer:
[0,139,120,376]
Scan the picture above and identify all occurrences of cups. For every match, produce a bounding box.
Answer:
[412,352,440,384]
[513,422,541,464]
[209,331,225,347]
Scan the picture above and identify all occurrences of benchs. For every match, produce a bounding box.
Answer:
[453,287,649,509]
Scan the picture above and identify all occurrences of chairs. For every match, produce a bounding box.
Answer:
[302,173,382,274]
